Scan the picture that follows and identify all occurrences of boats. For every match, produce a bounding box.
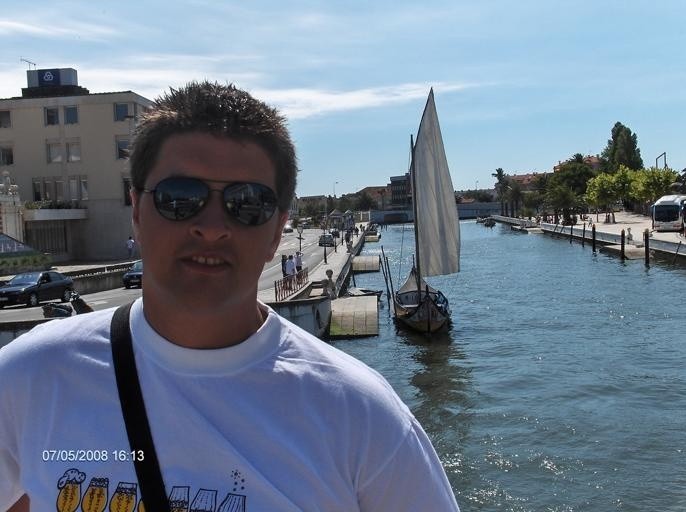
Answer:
[475,216,495,228]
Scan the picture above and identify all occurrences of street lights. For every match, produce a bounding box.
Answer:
[295,224,302,251]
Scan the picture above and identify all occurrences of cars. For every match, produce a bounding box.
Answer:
[121,261,143,289]
[282,225,292,232]
[318,228,338,246]
[0,271,74,310]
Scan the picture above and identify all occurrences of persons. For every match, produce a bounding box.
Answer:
[292,250,304,274]
[125,236,135,260]
[0,74,463,510]
[345,231,350,244]
[281,254,288,290]
[354,227,359,234]
[284,255,298,290]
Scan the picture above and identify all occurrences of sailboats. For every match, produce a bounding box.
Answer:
[392,87,460,335]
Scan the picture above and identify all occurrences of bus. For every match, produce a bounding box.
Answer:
[650,194,685,231]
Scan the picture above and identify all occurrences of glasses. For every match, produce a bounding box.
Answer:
[142,176,282,228]
